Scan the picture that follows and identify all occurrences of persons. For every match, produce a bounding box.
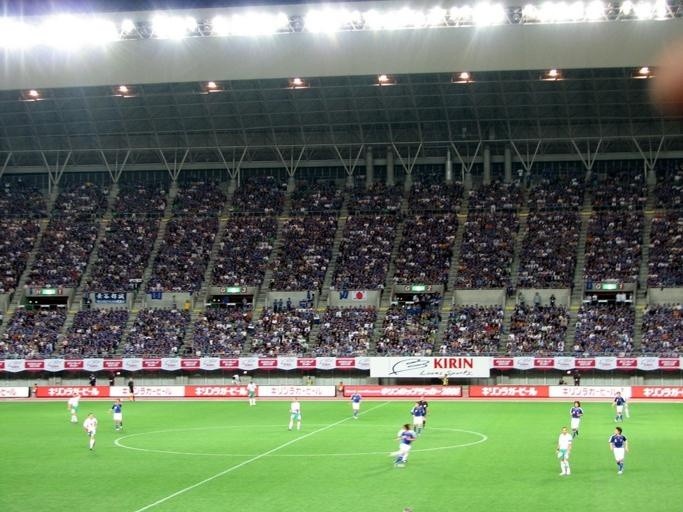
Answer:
[555,428,572,475]
[89,373,96,386]
[608,428,628,477]
[305,376,313,386]
[388,425,414,466]
[559,371,580,386]
[232,373,256,406]
[112,399,123,430]
[442,374,448,386]
[32,383,37,397]
[128,378,135,402]
[337,381,344,392]
[351,391,362,418]
[570,401,583,435]
[82,413,97,450]
[612,392,626,422]
[411,396,428,434]
[68,392,79,423]
[109,373,115,386]
[288,397,301,431]
[0,169,682,359]
